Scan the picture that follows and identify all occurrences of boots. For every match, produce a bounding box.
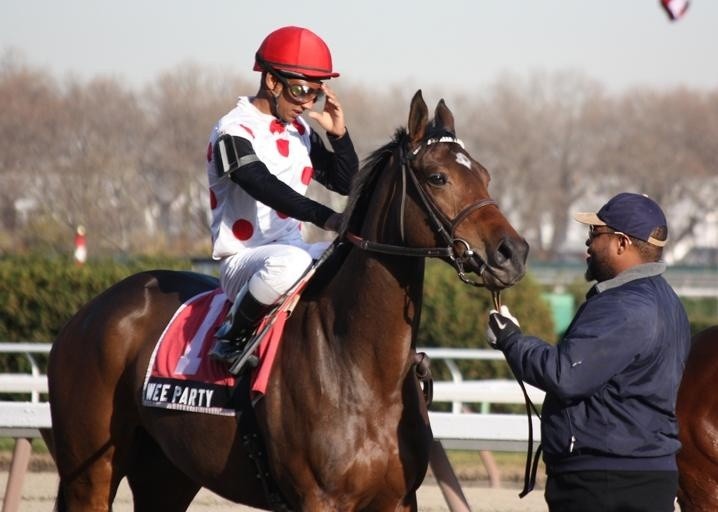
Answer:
[205,274,283,369]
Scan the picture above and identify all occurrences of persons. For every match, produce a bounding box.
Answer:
[205,26,359,376]
[484,193,696,512]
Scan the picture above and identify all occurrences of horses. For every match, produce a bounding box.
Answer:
[672,323,717,511]
[44,88,533,512]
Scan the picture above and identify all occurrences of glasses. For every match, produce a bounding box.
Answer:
[284,74,325,104]
[586,225,633,246]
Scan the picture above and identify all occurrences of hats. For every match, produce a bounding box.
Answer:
[573,192,671,248]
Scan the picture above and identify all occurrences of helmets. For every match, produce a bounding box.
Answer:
[253,24,341,80]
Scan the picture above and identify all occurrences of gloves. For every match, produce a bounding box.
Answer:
[484,301,522,350]
